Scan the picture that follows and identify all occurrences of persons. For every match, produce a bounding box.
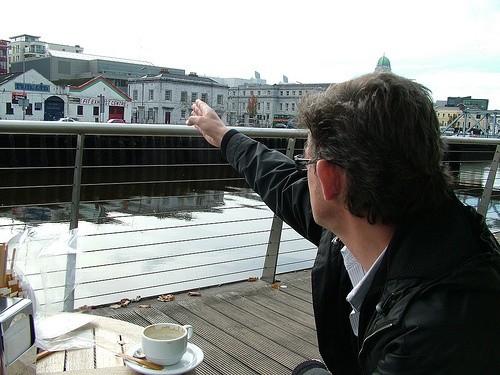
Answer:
[187,71,500,375]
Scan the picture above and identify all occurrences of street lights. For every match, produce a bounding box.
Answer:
[133,66,147,124]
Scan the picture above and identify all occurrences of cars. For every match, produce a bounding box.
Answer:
[107,118,124,124]
[58,118,77,122]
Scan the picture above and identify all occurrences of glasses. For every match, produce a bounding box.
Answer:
[294,154,346,174]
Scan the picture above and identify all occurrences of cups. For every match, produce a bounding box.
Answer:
[142,322,193,365]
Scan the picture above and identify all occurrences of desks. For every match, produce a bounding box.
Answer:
[35,312,144,375]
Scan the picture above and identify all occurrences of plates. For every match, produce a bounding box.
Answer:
[124,342,204,375]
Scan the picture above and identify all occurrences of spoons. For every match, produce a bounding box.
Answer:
[118,352,163,370]
